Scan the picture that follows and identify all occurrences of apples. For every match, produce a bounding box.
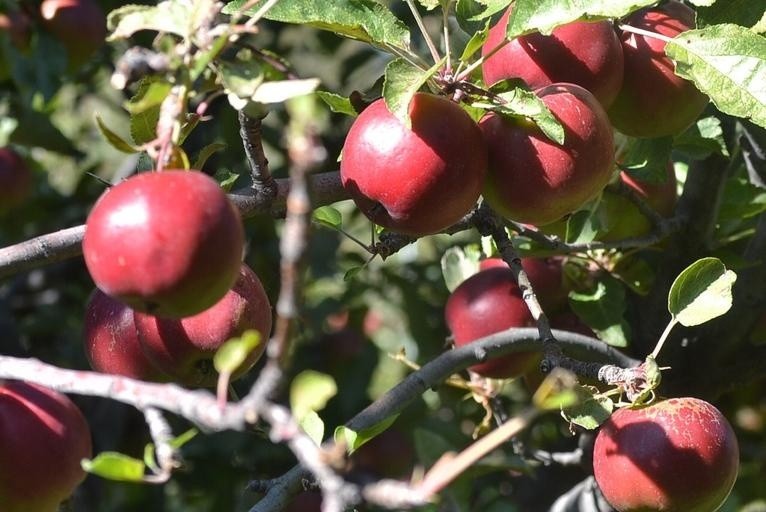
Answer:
[0,148,30,217]
[286,430,392,512]
[82,168,273,388]
[0,382,93,512]
[0,0,106,67]
[322,0,712,379]
[593,397,740,512]
